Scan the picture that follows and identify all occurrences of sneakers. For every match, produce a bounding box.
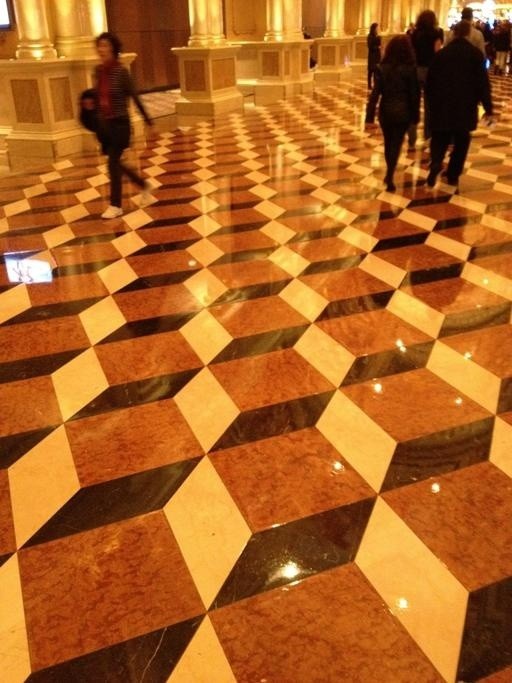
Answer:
[383,175,396,191]
[407,141,416,153]
[100,204,123,219]
[428,167,441,186]
[140,182,153,206]
[446,176,458,185]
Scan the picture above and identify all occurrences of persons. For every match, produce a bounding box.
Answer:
[92,31,155,219]
[364,6,512,193]
